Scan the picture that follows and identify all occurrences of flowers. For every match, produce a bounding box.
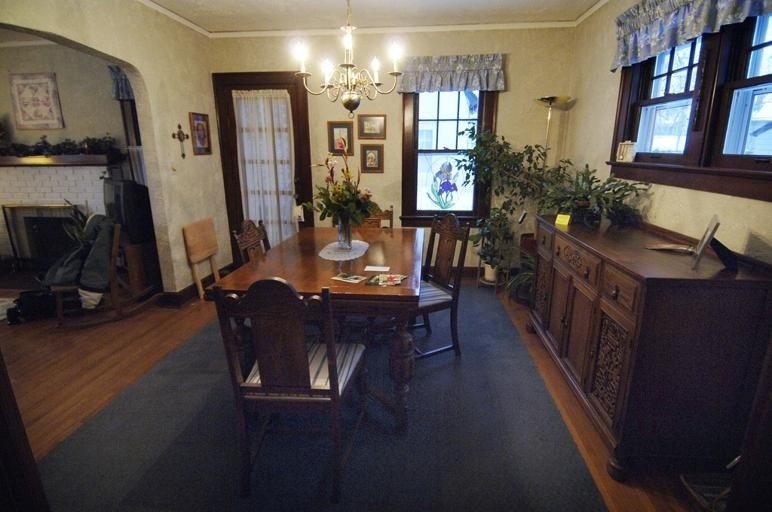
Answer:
[299,152,381,227]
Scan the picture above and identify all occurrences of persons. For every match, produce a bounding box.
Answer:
[196,122,206,146]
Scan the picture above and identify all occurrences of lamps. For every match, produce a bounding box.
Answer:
[294,1,401,120]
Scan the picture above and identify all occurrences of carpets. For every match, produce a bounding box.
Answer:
[36,278,610,512]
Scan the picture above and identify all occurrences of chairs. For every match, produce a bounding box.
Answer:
[50,224,122,333]
[232,218,271,266]
[407,213,471,357]
[212,276,371,503]
[354,201,393,228]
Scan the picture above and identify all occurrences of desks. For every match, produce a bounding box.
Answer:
[204,226,424,426]
[183,217,221,300]
[117,241,162,305]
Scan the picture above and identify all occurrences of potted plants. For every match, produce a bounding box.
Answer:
[456,123,652,305]
[468,207,517,282]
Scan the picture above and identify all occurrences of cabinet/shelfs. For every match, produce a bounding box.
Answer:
[526,212,772,483]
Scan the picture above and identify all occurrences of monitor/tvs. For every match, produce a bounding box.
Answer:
[102,177,156,243]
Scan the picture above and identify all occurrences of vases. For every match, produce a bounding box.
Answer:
[338,216,352,251]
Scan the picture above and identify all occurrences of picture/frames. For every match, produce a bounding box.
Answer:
[357,114,386,140]
[616,141,639,163]
[327,121,354,157]
[189,112,212,155]
[691,213,738,272]
[360,143,385,174]
